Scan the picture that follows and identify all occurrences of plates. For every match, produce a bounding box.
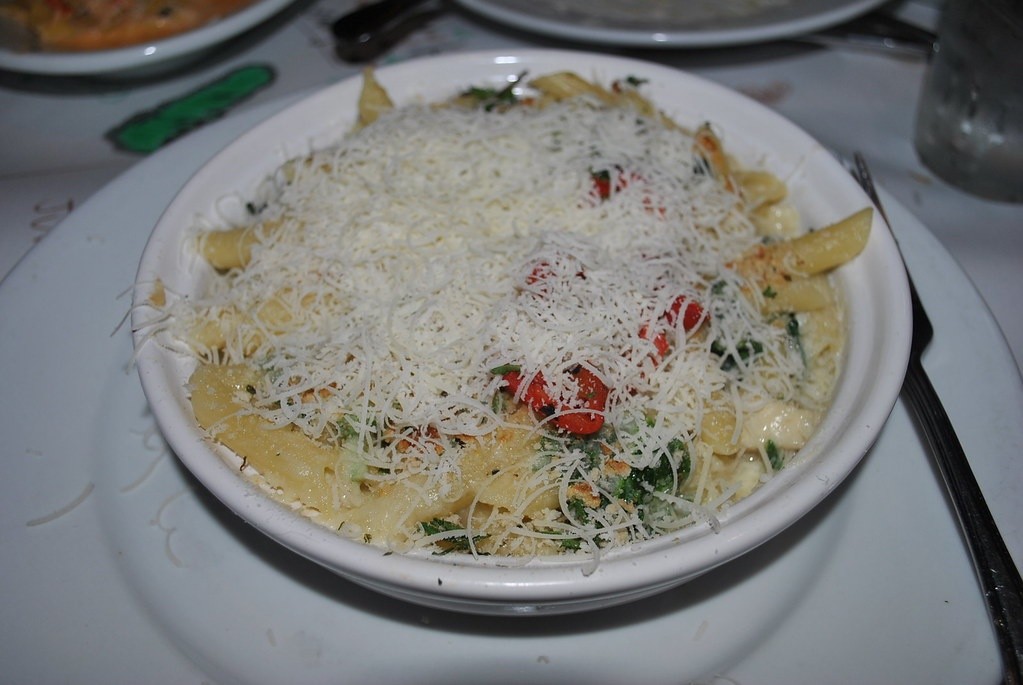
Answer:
[131,49,913,617]
[0,0,295,79]
[0,80,1023,685]
[460,0,883,49]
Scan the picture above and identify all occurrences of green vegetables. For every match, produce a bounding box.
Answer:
[245,67,809,559]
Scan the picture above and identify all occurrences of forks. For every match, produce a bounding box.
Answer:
[831,148,1023,685]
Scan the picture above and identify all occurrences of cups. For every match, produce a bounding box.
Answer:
[912,0,1023,202]
